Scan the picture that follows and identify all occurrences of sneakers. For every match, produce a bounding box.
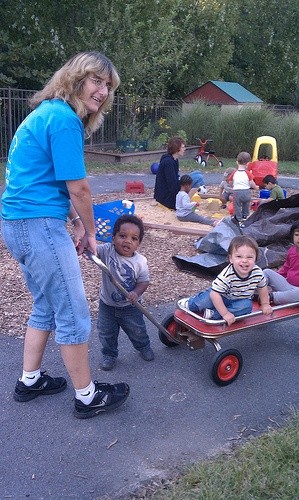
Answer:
[13,370,67,403]
[73,379,131,419]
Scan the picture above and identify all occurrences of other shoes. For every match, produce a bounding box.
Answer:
[221,204,226,209]
[179,297,189,310]
[101,356,115,370]
[242,215,247,220]
[138,343,154,360]
[202,307,214,319]
[258,293,274,306]
[252,293,258,300]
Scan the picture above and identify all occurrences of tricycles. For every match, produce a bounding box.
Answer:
[194,137,224,168]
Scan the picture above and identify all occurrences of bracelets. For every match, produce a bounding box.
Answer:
[71,216,80,221]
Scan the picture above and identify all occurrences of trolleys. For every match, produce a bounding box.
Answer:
[70,243,299,386]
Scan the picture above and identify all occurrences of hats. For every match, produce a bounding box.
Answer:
[221,167,236,181]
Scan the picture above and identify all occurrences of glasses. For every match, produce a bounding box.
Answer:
[88,75,112,89]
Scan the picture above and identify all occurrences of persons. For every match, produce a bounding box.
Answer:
[254,220,299,305]
[181,236,273,326]
[0,50,130,420]
[218,167,237,209]
[176,175,219,227]
[96,214,155,371]
[263,175,286,204]
[154,137,205,211]
[228,152,259,221]
